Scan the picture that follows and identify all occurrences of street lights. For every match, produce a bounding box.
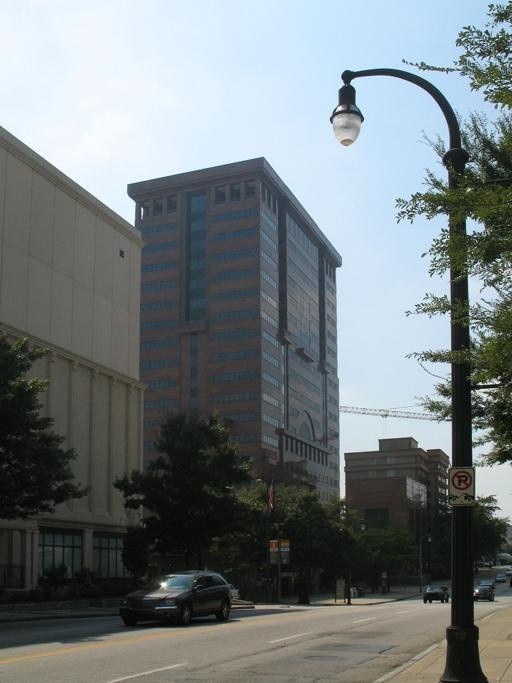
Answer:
[331,64,492,683]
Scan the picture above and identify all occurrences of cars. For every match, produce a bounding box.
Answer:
[423,567,512,603]
[119,569,234,626]
